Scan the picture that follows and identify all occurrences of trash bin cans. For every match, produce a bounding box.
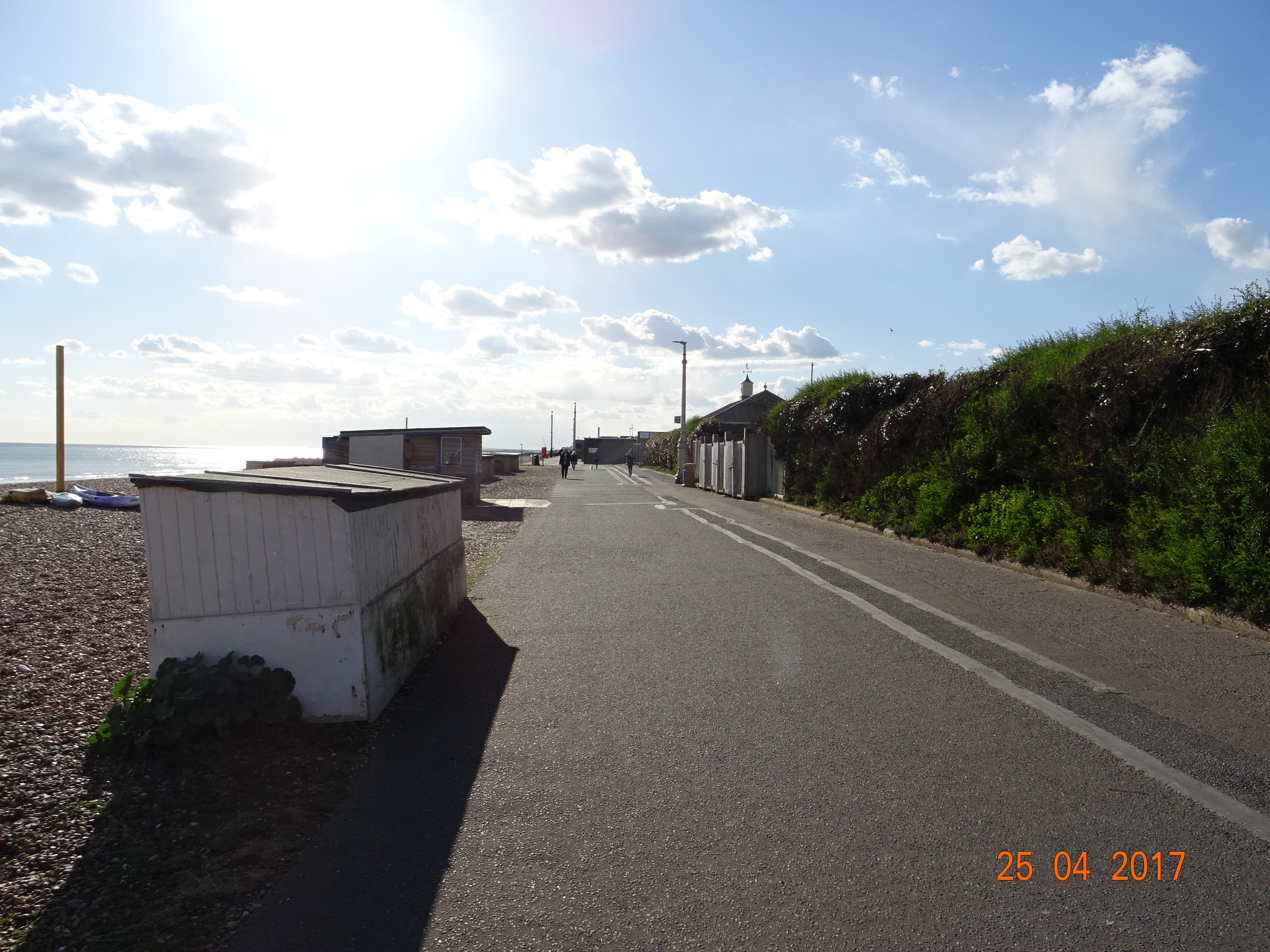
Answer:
[681,462,695,487]
[546,453,548,458]
[532,454,539,465]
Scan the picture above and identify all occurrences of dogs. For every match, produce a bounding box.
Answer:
[590,465,593,470]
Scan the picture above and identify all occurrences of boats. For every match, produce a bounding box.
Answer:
[1,488,56,503]
[74,483,140,510]
[51,491,83,508]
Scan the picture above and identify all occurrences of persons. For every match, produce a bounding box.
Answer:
[551,448,578,479]
[624,448,635,476]
[594,448,600,470]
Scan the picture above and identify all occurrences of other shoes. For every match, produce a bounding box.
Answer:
[630,471,632,474]
[565,476,567,479]
[573,469,575,470]
[562,476,564,479]
[629,474,630,477]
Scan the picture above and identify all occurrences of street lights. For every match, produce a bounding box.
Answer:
[672,340,690,484]
[573,403,576,451]
[520,444,522,466]
